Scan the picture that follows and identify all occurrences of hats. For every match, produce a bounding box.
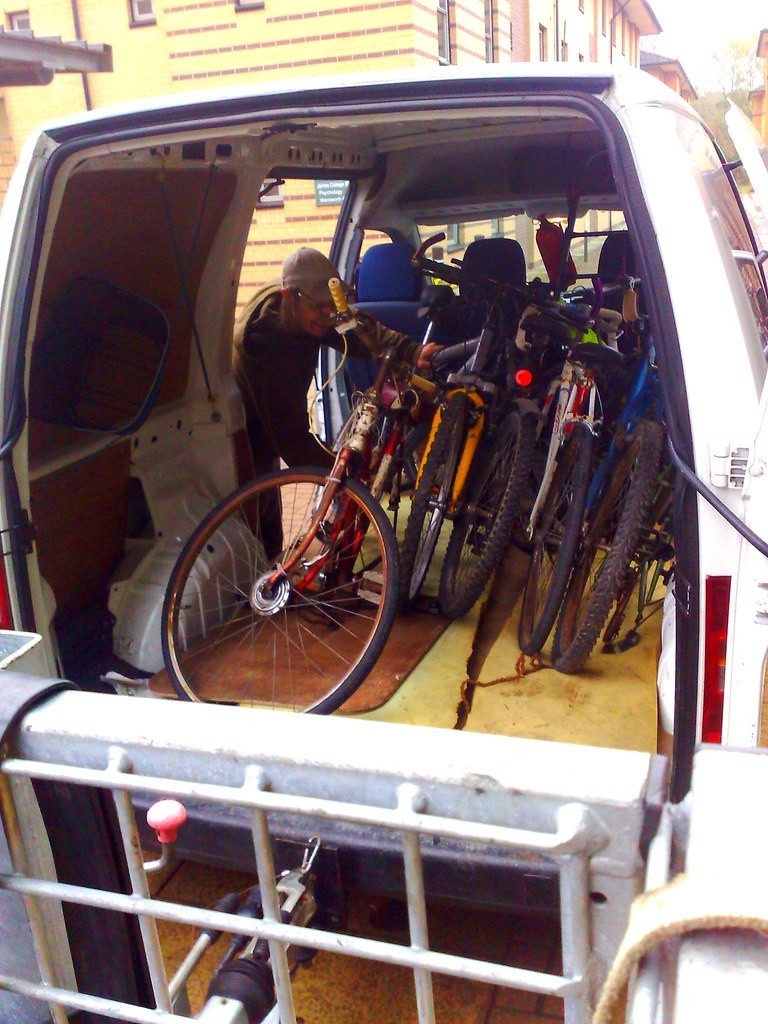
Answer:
[283,247,351,302]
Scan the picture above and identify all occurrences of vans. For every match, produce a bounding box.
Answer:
[4,60,768,923]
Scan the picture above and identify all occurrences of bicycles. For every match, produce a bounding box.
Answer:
[160,230,667,716]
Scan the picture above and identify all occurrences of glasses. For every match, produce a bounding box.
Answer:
[289,288,340,310]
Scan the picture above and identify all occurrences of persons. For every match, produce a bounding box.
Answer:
[233,246,442,565]
